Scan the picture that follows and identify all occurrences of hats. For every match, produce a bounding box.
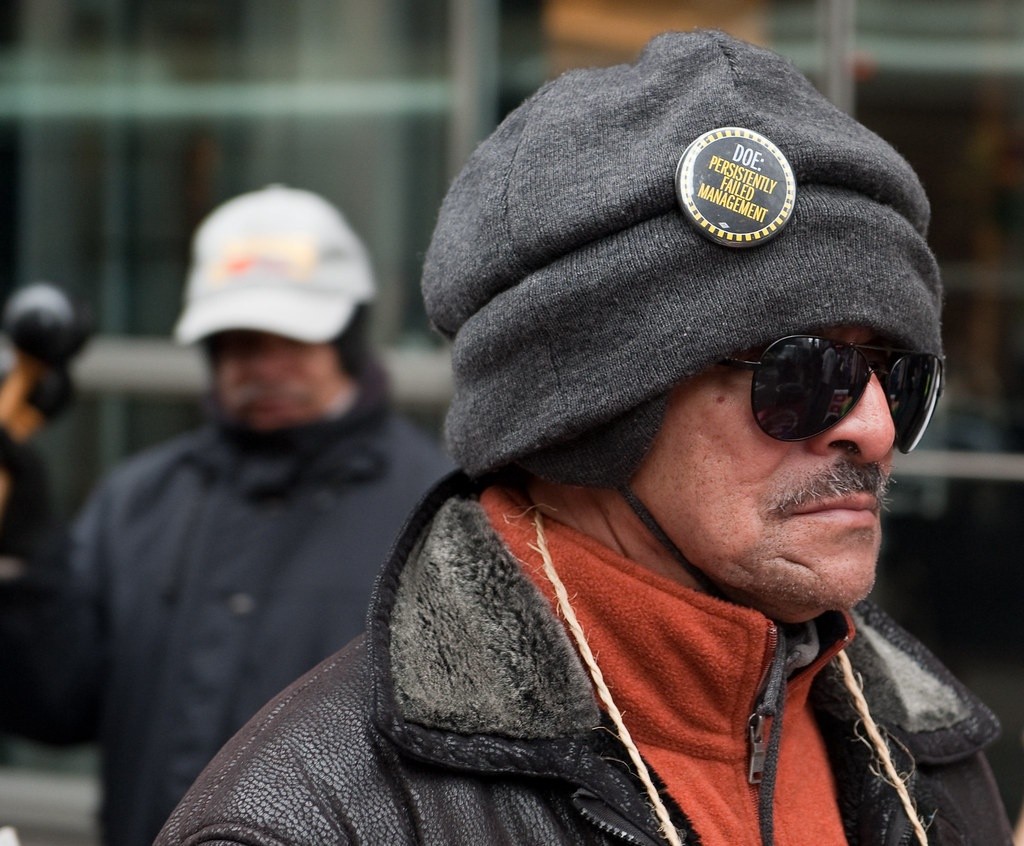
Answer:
[167,180,374,346]
[419,25,946,490]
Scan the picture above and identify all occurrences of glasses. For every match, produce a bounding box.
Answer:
[715,334,939,455]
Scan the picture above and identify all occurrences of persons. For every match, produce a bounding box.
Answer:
[157,33,1005,846]
[0,182,465,846]
